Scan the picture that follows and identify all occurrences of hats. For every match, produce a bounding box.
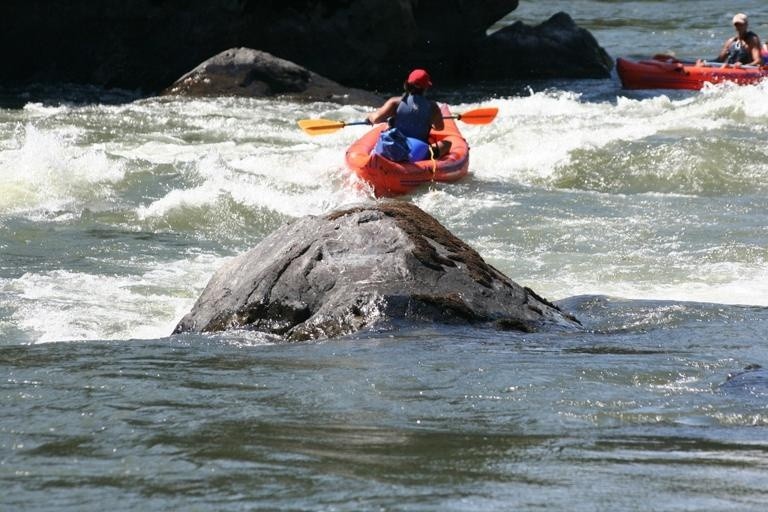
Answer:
[732,13,749,26]
[407,68,433,89]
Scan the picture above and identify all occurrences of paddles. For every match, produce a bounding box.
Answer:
[653,54,768,78]
[298,108,498,135]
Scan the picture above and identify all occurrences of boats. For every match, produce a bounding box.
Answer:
[616,56,768,90]
[344,103,469,200]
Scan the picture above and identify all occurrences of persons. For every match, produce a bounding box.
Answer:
[368,69,452,166]
[694,12,768,71]
